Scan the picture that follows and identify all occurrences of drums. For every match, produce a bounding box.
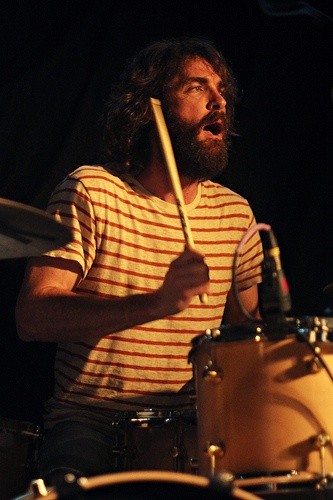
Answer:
[186,317,333,481]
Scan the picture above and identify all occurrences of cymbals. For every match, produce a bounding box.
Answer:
[1,196,74,257]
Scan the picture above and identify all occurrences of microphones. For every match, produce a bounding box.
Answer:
[258,224,293,317]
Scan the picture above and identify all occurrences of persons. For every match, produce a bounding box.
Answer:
[15,39,265,468]
